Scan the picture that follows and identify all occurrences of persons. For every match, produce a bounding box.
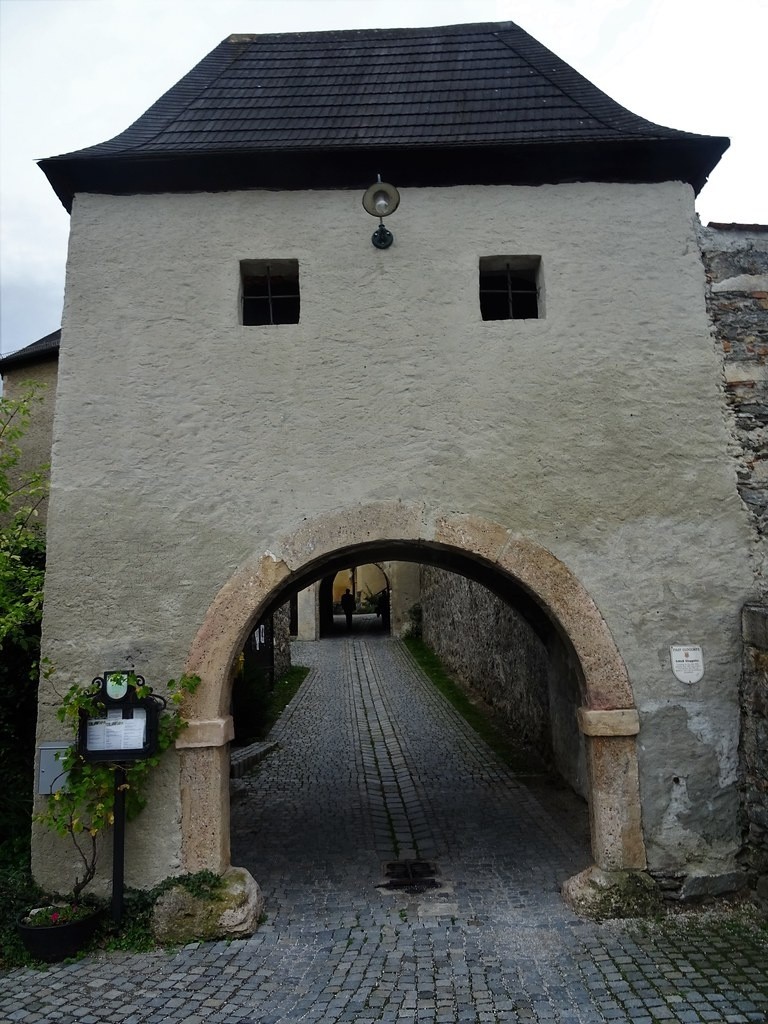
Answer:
[377,590,390,622]
[341,589,357,623]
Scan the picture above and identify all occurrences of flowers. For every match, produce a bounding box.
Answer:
[23,903,94,928]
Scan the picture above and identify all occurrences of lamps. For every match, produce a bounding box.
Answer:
[363,174,400,251]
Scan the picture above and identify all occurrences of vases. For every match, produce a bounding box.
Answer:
[17,904,101,963]
